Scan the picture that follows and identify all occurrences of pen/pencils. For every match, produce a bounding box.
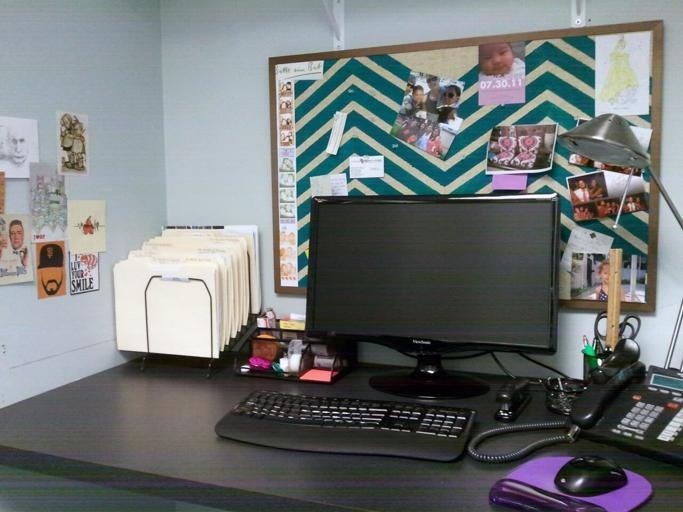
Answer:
[582,335,599,378]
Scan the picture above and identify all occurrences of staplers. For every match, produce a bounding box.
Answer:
[494,379,531,422]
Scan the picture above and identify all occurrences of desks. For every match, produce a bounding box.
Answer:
[0,337,683,512]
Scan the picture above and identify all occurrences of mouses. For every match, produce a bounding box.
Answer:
[553,454,628,497]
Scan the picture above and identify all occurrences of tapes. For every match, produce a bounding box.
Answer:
[289,353,302,372]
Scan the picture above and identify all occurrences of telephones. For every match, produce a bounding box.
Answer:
[571,361,683,465]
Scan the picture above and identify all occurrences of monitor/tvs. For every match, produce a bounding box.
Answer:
[305,193,561,400]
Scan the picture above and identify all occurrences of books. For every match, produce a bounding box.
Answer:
[112,223,261,361]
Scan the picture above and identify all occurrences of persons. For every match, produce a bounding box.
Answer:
[478,42,526,80]
[594,260,625,301]
[0,124,34,168]
[562,157,646,222]
[0,219,29,274]
[394,74,461,160]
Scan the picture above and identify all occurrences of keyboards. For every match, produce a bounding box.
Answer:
[214,390,478,463]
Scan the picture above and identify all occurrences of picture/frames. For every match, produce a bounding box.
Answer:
[266,18,664,315]
